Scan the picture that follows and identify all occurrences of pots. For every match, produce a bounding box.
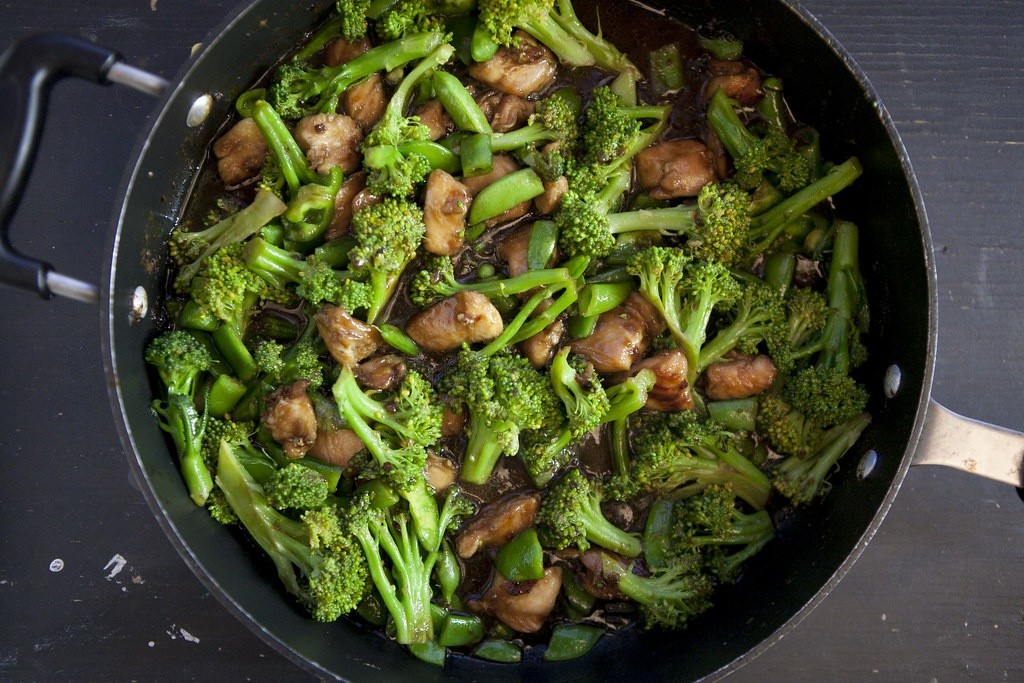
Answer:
[0,0,1024,683]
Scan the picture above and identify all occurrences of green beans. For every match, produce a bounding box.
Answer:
[359,536,607,663]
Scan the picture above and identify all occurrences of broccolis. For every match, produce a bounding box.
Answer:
[141,0,873,644]
